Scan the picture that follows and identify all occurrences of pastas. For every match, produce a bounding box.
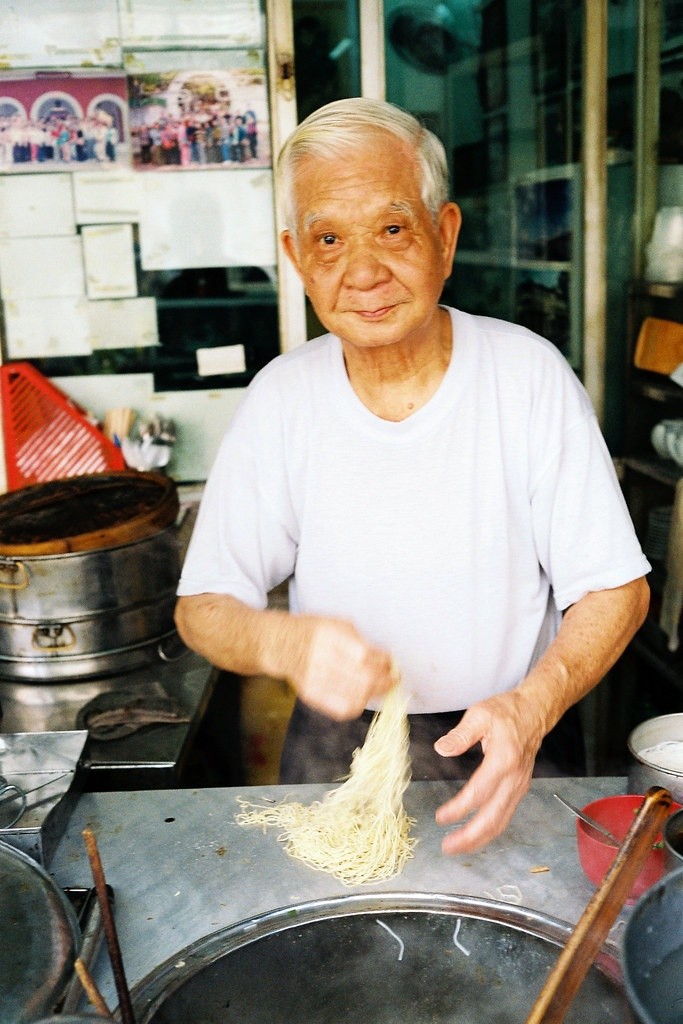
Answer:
[234,668,422,886]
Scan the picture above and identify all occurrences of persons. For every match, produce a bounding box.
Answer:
[0,91,259,165]
[172,97,653,859]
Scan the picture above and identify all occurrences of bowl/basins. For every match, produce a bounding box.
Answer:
[577,795,682,904]
[663,807,683,870]
[617,867,683,1024]
[627,713,683,805]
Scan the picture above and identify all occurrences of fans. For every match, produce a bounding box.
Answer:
[385,3,481,77]
